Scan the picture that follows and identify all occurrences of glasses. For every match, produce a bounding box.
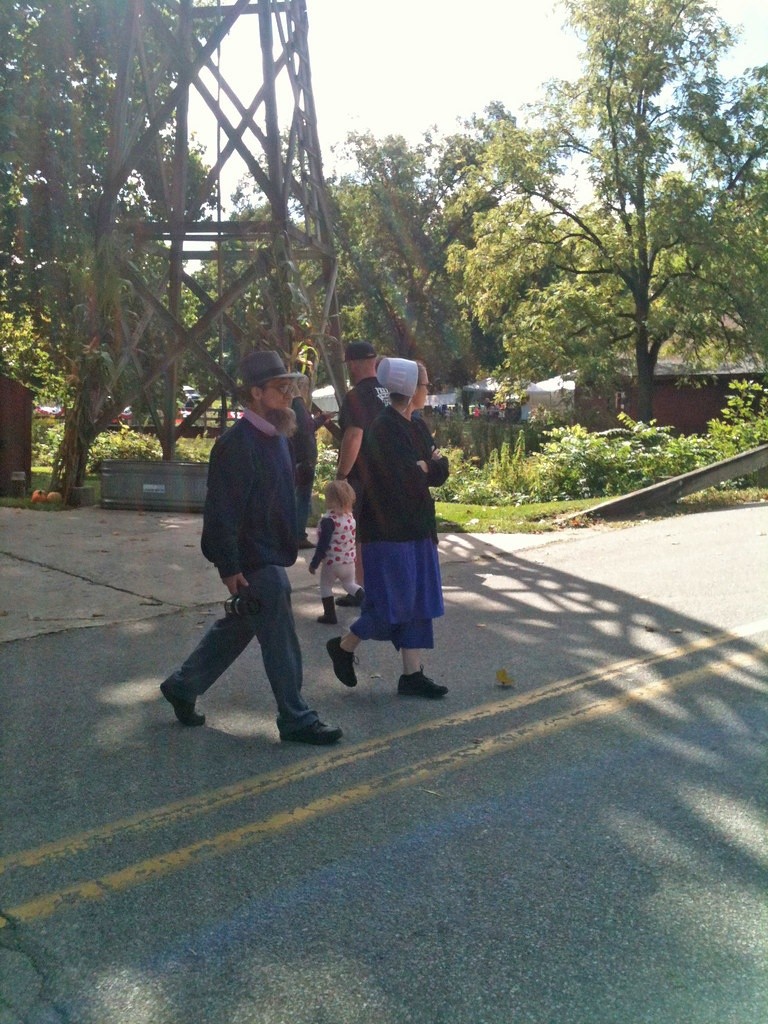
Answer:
[260,383,295,395]
[416,382,434,393]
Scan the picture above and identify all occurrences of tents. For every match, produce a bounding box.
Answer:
[464,377,550,412]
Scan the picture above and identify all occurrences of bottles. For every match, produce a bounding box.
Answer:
[224,589,260,617]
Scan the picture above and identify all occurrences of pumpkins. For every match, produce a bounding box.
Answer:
[32,489,62,503]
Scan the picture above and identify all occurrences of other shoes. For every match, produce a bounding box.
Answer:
[336,593,361,607]
[296,540,315,551]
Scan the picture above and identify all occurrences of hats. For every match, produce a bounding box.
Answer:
[341,341,376,364]
[376,358,418,399]
[236,350,306,391]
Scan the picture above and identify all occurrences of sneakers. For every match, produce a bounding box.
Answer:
[160,682,206,726]
[280,721,342,745]
[397,664,449,696]
[326,636,358,687]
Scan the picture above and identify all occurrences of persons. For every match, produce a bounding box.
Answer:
[160,350,344,744]
[500,403,504,411]
[185,396,194,411]
[474,398,490,416]
[292,372,336,548]
[326,358,448,699]
[309,479,365,624]
[454,403,461,412]
[335,341,390,606]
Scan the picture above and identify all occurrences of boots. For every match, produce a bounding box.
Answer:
[355,586,366,603]
[317,596,337,624]
